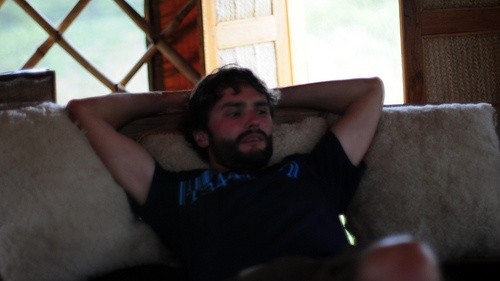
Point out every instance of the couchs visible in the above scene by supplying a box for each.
[1,96,500,281]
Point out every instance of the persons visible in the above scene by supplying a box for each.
[65,62,443,280]
